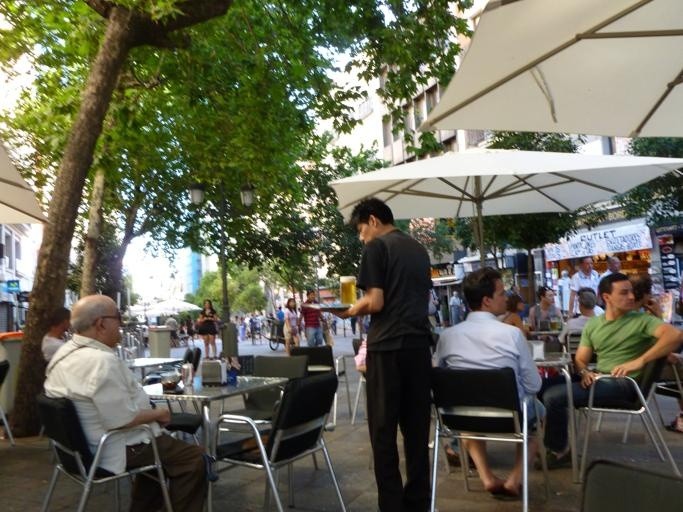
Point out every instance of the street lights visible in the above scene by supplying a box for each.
[187,174,254,358]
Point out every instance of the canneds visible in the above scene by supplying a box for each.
[181,362,194,386]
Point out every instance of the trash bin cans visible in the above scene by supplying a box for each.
[148,325,174,357]
[0,332,25,421]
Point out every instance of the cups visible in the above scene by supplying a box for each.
[550,315,560,332]
[160,371,179,392]
[339,276,356,303]
[522,317,529,330]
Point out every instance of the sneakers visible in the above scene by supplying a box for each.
[535,447,572,470]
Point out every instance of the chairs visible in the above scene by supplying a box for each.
[576,355,683,484]
[217,355,322,504]
[427,364,552,512]
[218,370,349,512]
[32,395,174,511]
[288,344,351,431]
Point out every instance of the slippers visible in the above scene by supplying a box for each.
[489,483,523,501]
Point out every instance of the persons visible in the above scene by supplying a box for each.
[41,288,371,361]
[531,272,682,472]
[662,390,682,438]
[430,266,547,497]
[428,257,682,361]
[326,196,432,512]
[42,295,209,511]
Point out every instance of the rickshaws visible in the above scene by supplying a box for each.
[257,318,294,350]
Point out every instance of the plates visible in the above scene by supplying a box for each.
[661,245,677,288]
[304,300,347,312]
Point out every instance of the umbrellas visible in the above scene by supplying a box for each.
[326,146,682,269]
[411,1,683,142]
